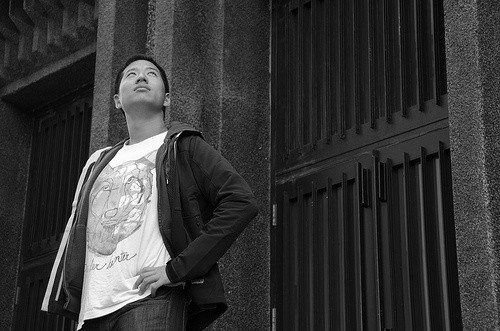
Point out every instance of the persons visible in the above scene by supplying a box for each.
[42,55,257,331]
